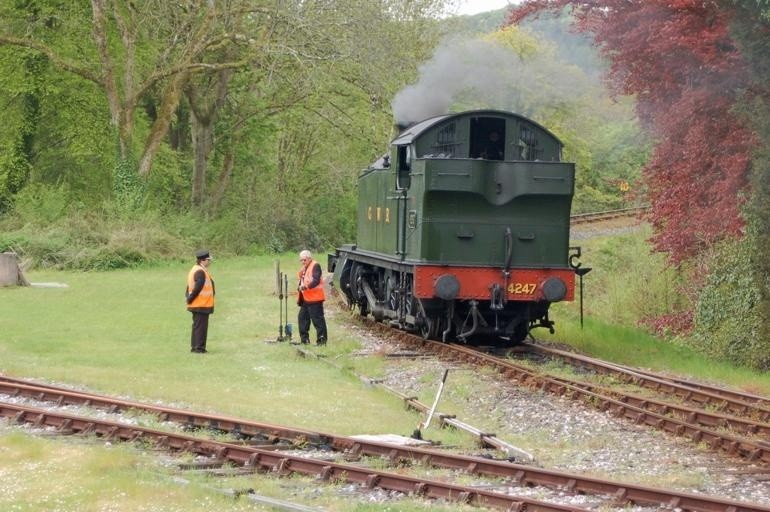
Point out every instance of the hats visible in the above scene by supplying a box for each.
[195,250,213,260]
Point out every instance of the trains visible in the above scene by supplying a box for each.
[324,110,593,345]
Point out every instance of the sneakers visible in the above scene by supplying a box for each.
[191,349,208,353]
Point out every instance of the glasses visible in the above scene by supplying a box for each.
[300,259,306,261]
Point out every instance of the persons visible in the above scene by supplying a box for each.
[183,249,216,354]
[294,249,328,346]
